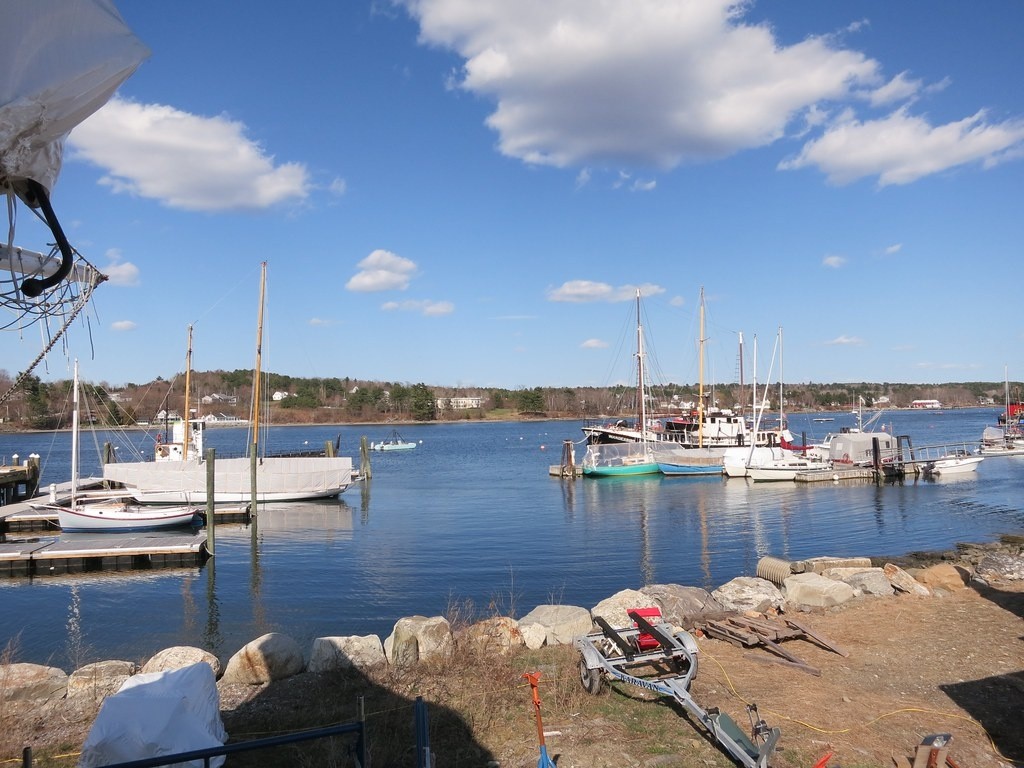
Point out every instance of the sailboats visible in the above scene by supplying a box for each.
[979,362,1024,457]
[652,286,800,475]
[580,286,794,448]
[851,390,858,414]
[103,260,352,502]
[25,358,205,533]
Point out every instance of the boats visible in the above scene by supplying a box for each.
[996,401,1024,427]
[147,420,341,458]
[374,429,416,450]
[813,418,834,421]
[744,427,897,482]
[583,442,685,476]
[867,435,985,474]
